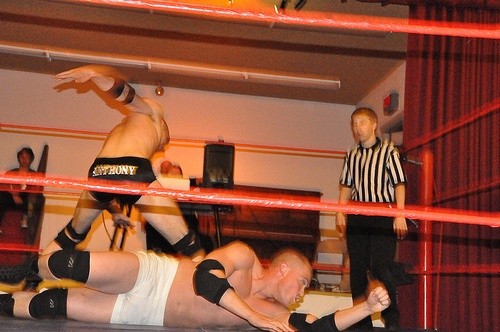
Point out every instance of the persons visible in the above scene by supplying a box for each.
[0,240,392,332]
[0,66,212,261]
[336,106,409,332]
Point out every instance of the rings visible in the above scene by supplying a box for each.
[387,299,390,303]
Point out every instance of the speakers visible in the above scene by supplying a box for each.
[202,144,235,190]
